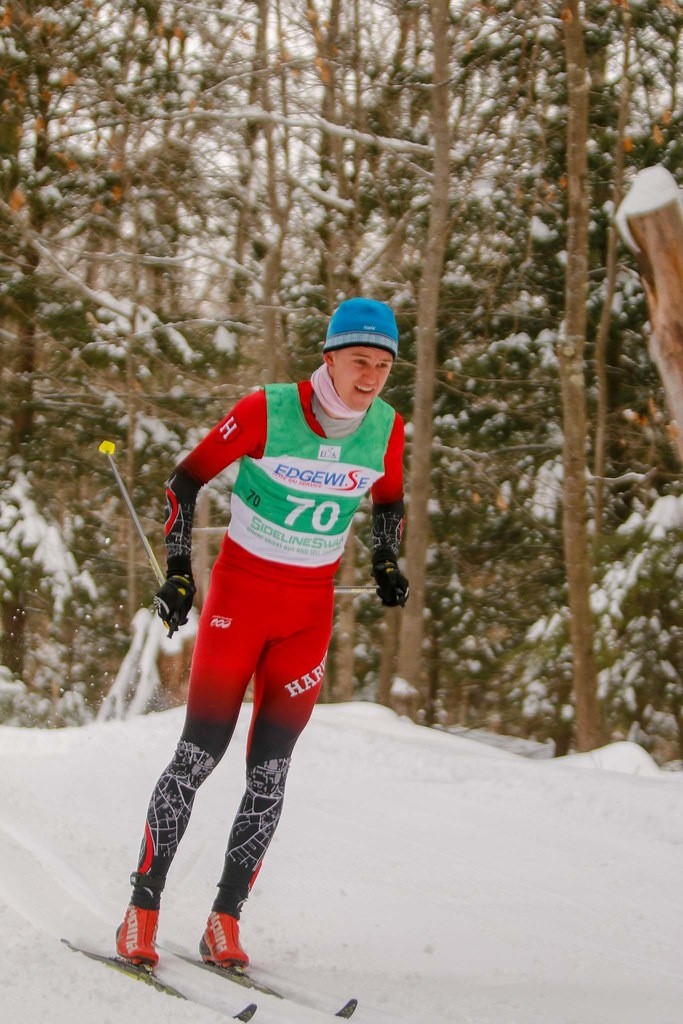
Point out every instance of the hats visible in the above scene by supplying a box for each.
[322,298,398,361]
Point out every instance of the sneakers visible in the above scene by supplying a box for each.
[116,903,160,968]
[200,911,249,969]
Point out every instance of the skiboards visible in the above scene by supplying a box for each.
[61,933,359,1024]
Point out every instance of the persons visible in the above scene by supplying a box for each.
[114,296,412,966]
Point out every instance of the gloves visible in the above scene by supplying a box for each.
[153,574,197,633]
[369,560,410,608]
